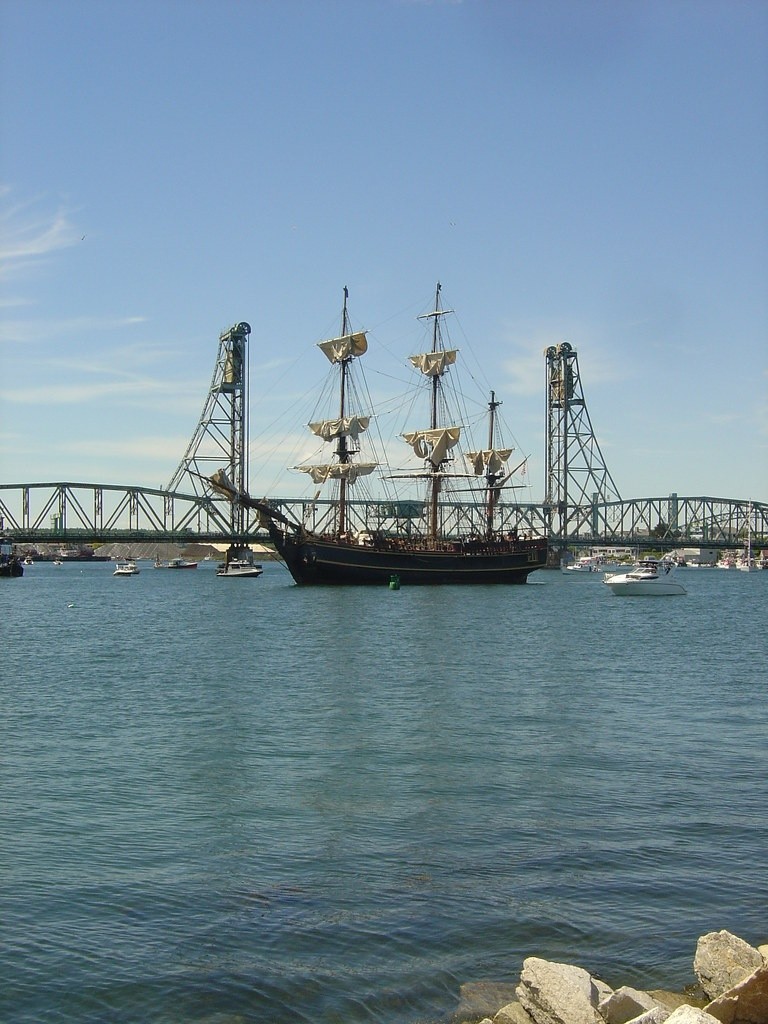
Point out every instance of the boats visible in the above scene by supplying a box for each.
[602,560,687,595]
[567,556,616,573]
[20,553,265,577]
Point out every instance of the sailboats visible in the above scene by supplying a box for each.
[717,497,760,572]
[184,280,550,585]
